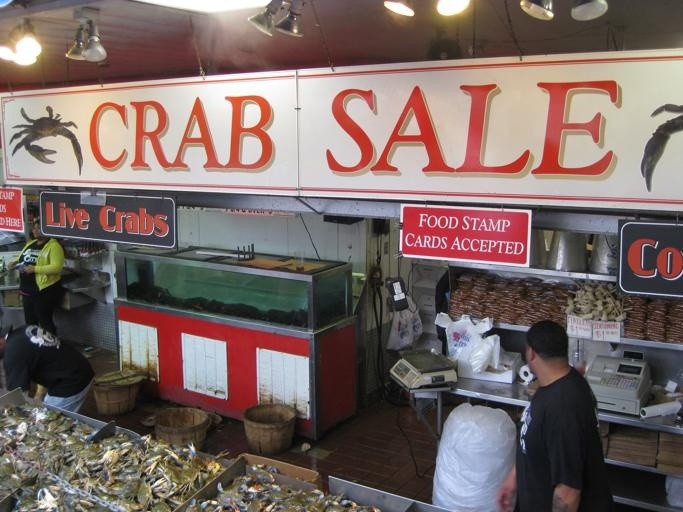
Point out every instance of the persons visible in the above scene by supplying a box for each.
[434,266,468,357]
[0,326,94,413]
[494,320,614,512]
[8,219,64,336]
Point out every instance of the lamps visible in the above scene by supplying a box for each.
[247,0,306,38]
[382,0,471,19]
[0,19,43,66]
[520,0,609,21]
[64,7,108,62]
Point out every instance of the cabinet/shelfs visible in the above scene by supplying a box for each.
[446,258,683,354]
[432,372,683,511]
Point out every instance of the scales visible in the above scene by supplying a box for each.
[389,351,458,389]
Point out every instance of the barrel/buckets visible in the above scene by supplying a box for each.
[94,382,139,415]
[244,404,297,456]
[155,407,209,451]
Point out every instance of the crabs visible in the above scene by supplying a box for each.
[9,105,84,176]
[187,462,380,512]
[640,103,683,192]
[0,400,228,512]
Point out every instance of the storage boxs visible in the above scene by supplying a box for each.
[169,451,324,511]
[457,346,522,384]
